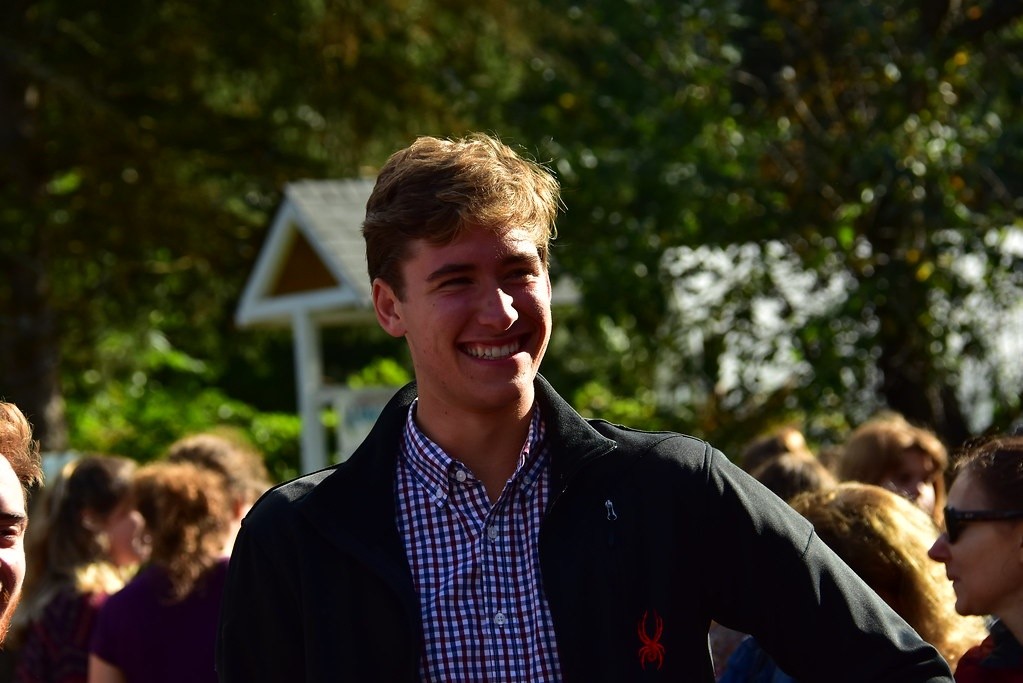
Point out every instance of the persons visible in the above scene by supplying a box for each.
[0,402,271,683]
[711,409,1023,683]
[214,131,954,683]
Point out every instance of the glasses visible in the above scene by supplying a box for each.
[943,505,1022,544]
